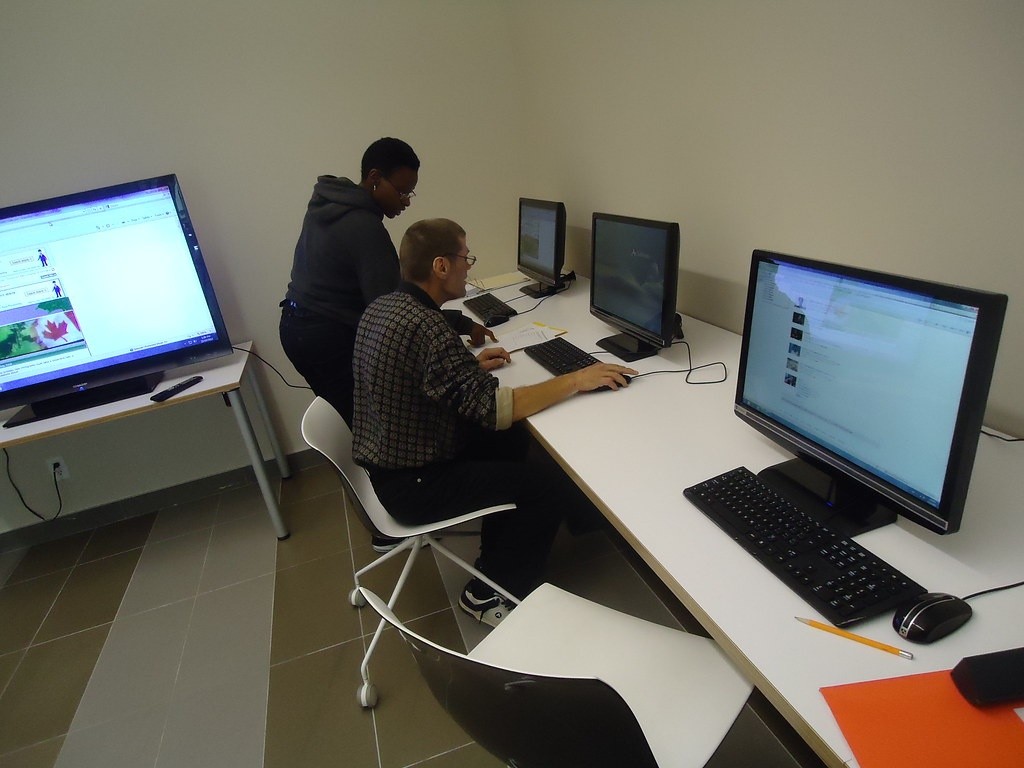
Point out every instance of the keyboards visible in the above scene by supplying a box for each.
[463,293,517,322]
[524,337,603,376]
[683,465,928,626]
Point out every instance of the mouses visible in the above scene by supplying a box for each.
[598,375,630,391]
[485,315,509,328]
[891,592,973,645]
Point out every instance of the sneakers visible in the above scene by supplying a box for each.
[457,577,517,628]
[371,536,443,552]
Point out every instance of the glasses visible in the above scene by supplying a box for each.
[443,252,477,266]
[381,174,417,200]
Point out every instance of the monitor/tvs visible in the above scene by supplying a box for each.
[589,212,680,362]
[0,173,233,429]
[733,249,1008,538]
[517,197,568,299]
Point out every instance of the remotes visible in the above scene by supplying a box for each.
[150,376,203,402]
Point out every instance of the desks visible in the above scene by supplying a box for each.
[0,340,291,542]
[438,267,1024,768]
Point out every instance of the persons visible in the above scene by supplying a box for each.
[279,136,440,554]
[352,219,639,630]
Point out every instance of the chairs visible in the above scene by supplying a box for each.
[358,582,754,768]
[302,396,522,708]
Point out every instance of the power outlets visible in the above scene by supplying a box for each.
[52,460,69,481]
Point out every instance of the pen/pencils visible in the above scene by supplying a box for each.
[794,616,914,660]
